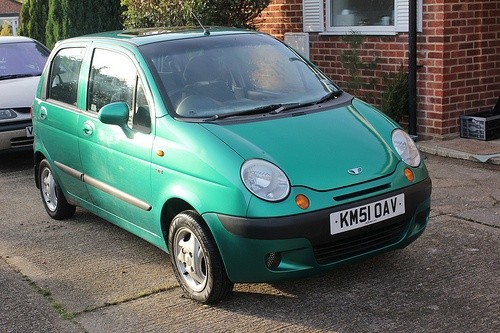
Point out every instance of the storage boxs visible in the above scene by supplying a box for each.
[460,109,500,141]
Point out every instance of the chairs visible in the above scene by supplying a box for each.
[183,53,238,103]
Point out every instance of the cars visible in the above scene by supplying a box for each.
[31,11,433,304]
[0,36,51,167]
